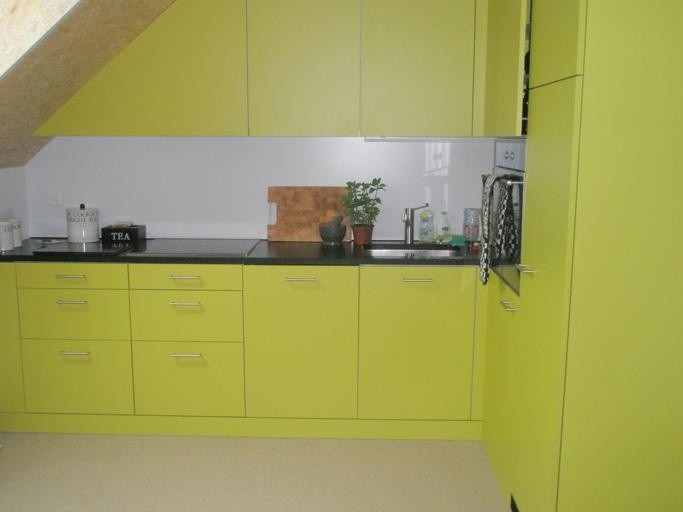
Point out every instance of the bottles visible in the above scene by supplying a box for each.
[437,211,449,235]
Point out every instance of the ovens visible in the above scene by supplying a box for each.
[486,165,527,298]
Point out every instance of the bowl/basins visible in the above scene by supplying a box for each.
[319,221,346,245]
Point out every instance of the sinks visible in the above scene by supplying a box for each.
[364,248,464,261]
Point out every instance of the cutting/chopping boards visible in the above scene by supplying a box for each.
[267,186,353,243]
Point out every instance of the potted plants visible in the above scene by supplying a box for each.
[341,177,388,247]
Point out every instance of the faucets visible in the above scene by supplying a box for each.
[405,202,429,245]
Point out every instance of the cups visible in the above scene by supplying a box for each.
[9,218,23,248]
[0,218,15,252]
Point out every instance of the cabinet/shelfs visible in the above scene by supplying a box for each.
[242,265,479,440]
[1,262,30,435]
[13,262,246,438]
[246,0,476,140]
[472,3,683,512]
[33,0,248,139]
